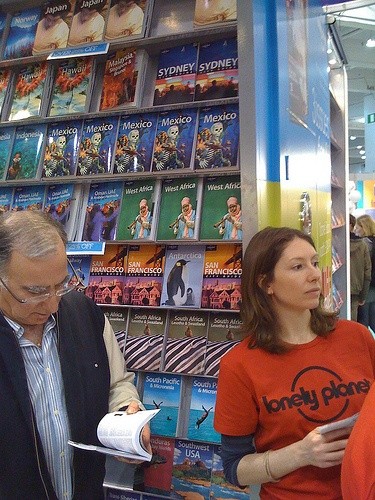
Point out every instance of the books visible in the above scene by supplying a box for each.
[67,408,160,462]
[0,0,250,500]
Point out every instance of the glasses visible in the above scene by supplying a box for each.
[2,259,83,305]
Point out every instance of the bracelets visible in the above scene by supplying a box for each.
[262,449,280,482]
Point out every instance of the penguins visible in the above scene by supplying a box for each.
[161,259,196,306]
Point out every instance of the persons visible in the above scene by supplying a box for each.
[131,198,153,239]
[32,0,71,55]
[68,0,107,45]
[219,197,243,240]
[0,210,166,469]
[173,197,196,239]
[350,214,371,321]
[213,227,375,500]
[355,215,375,333]
[105,0,144,40]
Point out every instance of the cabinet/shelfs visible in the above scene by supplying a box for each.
[1,0,349,499]
